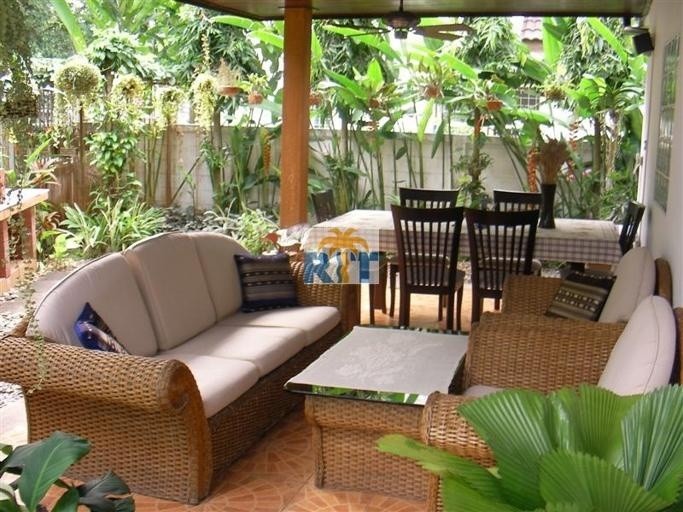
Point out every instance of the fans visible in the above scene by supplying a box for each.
[331,8,473,44]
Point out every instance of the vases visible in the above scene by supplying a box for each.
[538,183,557,229]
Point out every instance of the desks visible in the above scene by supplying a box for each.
[303,208,623,284]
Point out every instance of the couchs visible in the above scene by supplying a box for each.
[420,296,683,511]
[478,248,674,329]
[1,234,360,504]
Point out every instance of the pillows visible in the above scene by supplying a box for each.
[234,251,300,313]
[546,269,619,322]
[73,302,133,355]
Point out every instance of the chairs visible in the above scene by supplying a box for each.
[558,199,646,278]
[391,207,466,330]
[311,189,337,223]
[492,190,544,215]
[397,185,460,208]
[469,207,541,327]
